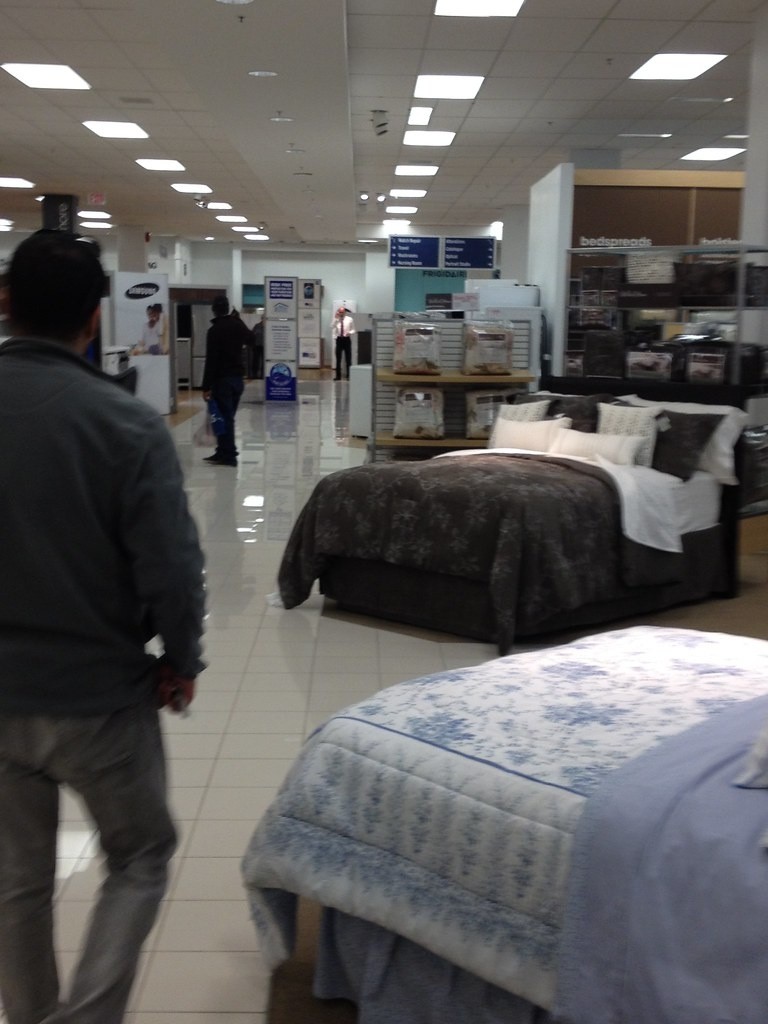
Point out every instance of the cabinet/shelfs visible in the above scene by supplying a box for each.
[364,314,545,460]
[565,244,768,516]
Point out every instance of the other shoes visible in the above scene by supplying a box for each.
[333,376,341,380]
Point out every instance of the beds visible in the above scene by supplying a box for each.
[275,388,754,658]
[235,622,767,1024]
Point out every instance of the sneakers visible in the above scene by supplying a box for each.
[207,458,238,466]
[202,454,221,460]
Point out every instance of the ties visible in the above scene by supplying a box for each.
[340,318,344,337]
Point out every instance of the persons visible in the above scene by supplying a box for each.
[200,294,255,467]
[0,226,207,1024]
[248,313,266,378]
[329,307,355,382]
[139,302,167,354]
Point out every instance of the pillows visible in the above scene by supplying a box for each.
[595,400,663,471]
[652,407,727,482]
[546,428,647,470]
[487,399,550,451]
[493,414,574,456]
[513,392,618,435]
